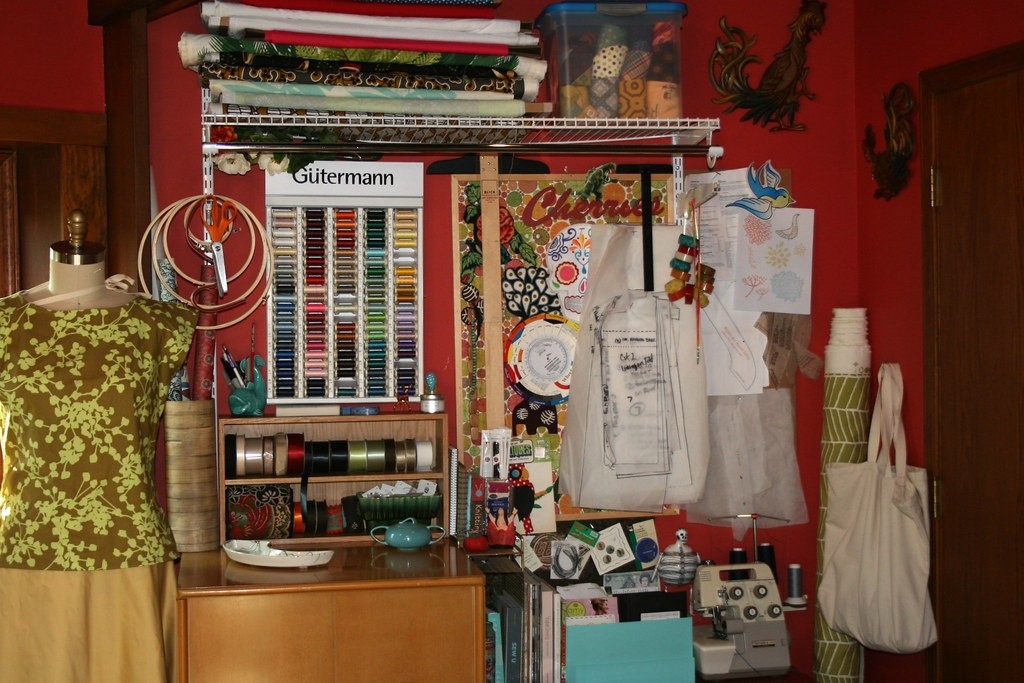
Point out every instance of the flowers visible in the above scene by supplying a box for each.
[209,125,289,175]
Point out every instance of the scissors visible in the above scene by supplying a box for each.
[200,193,238,297]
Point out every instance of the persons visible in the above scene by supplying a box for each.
[0,244,197,683]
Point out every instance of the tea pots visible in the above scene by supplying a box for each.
[369,517,446,551]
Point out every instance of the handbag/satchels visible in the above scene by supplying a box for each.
[816,364,938,654]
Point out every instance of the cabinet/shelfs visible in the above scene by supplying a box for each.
[218,413,449,547]
[175,550,488,683]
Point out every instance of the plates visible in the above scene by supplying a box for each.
[222,539,334,568]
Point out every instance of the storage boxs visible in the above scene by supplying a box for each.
[533,0,688,125]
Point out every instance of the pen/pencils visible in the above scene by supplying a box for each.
[221,321,256,392]
